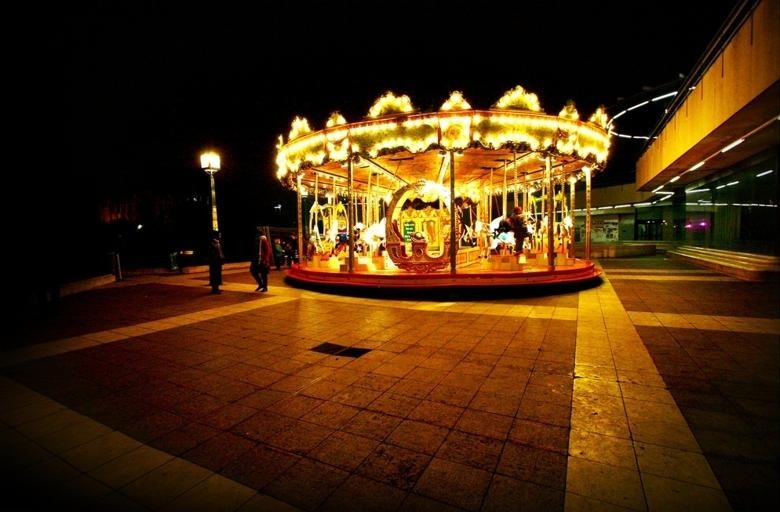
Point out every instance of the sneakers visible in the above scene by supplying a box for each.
[256,285,267,292]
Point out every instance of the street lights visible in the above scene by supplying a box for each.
[200,152,221,232]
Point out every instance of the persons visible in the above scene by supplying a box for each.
[510,207,533,254]
[249,225,307,292]
[205,231,224,295]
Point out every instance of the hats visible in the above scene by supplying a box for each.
[513,207,522,214]
